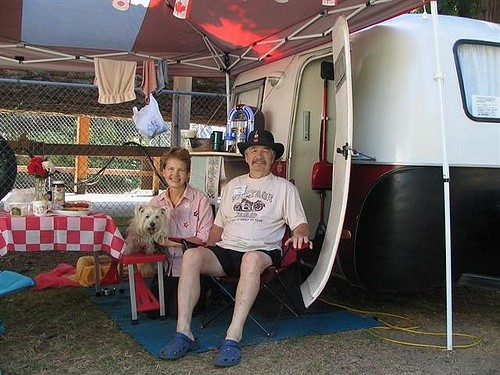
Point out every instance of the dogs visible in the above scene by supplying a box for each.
[117,204,172,281]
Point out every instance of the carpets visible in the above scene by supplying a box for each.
[83,277,384,361]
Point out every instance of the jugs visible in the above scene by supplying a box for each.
[210,131,223,151]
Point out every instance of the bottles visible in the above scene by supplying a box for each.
[224,137,236,152]
[52,181,65,207]
[235,128,246,154]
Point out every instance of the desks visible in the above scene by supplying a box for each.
[4,193,161,222]
[0,209,125,296]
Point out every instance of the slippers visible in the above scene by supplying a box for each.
[215,339,241,366]
[160,331,200,359]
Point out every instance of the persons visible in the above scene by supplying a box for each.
[150,147,214,321]
[159,130,314,367]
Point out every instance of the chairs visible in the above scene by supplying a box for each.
[179,180,309,339]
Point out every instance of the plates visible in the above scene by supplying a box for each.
[62,201,94,210]
[54,210,93,216]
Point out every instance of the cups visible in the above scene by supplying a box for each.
[32,200,52,216]
[10,203,31,216]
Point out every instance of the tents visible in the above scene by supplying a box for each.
[0,1,452,351]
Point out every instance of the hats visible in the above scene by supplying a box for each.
[237,129,284,160]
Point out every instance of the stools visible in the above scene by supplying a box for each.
[119,250,166,325]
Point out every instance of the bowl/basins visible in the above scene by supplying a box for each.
[180,130,198,138]
[3,202,24,212]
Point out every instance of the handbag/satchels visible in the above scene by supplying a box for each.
[131,93,169,141]
